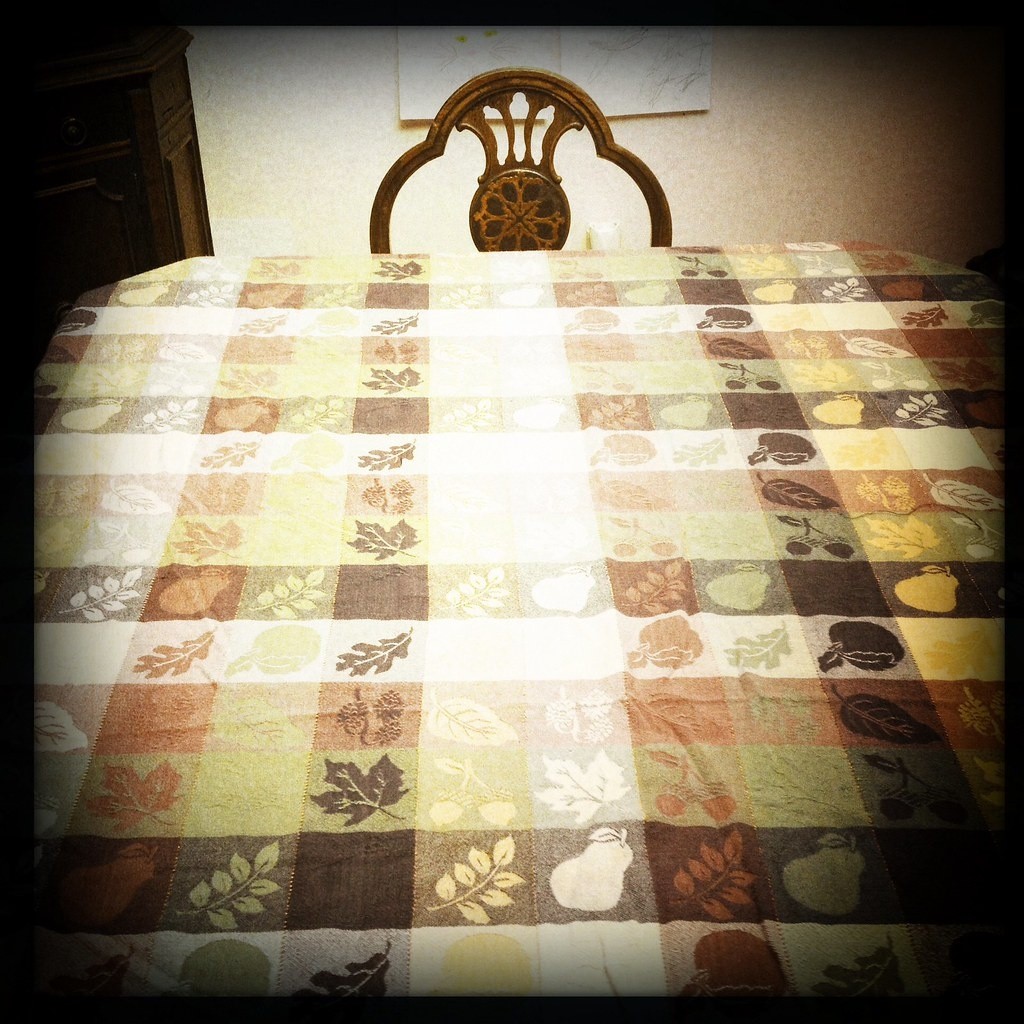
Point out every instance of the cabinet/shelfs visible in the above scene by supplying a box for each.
[8,23,218,356]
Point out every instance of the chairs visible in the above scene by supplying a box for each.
[369,69,673,253]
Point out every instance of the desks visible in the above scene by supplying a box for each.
[31,240,1007,996]
